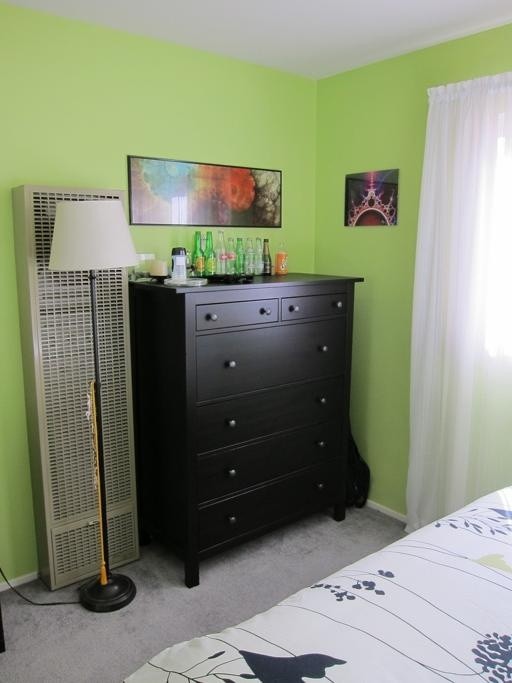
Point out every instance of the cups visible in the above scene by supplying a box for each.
[139,255,168,277]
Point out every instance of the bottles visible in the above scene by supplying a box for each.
[171,246,187,281]
[275,240,289,276]
[187,229,272,276]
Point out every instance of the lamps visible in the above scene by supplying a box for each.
[49,197,140,613]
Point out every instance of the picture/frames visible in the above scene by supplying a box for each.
[127,154,282,226]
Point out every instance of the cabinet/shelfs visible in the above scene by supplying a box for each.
[128,266,369,587]
